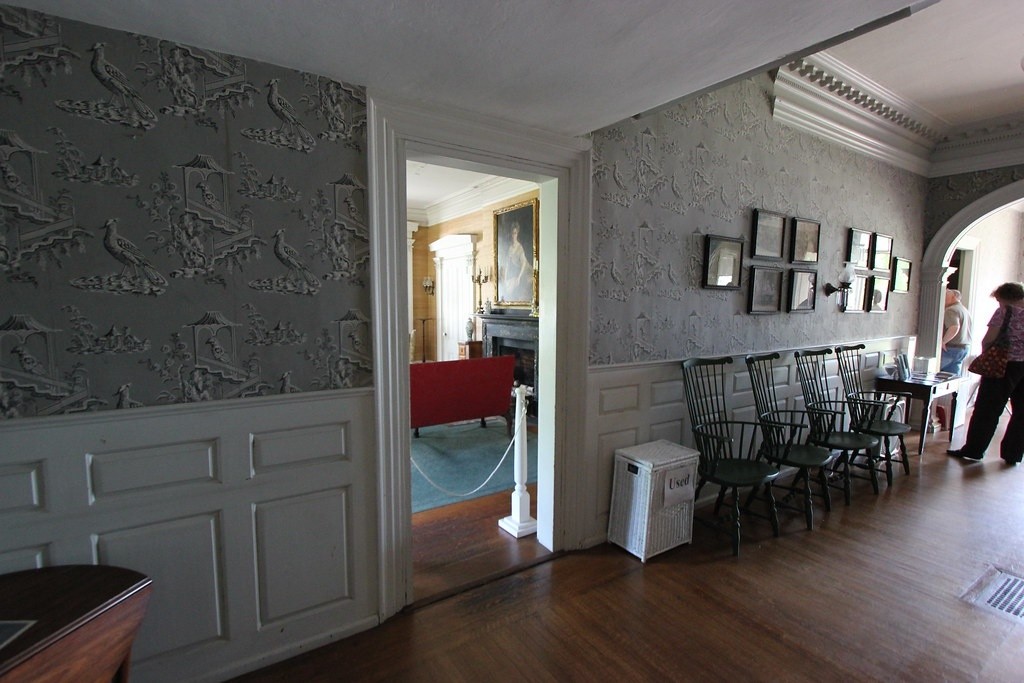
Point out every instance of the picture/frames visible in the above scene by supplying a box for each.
[847,228,872,269]
[839,274,868,313]
[788,270,818,313]
[791,217,821,265]
[869,275,892,312]
[492,196,540,306]
[891,257,912,294]
[703,233,744,290]
[873,232,893,271]
[753,209,787,260]
[747,266,785,315]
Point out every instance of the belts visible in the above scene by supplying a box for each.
[947,343,967,348]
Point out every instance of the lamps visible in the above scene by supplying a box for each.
[422,276,434,295]
[826,265,851,296]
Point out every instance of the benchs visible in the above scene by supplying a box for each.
[408,355,516,439]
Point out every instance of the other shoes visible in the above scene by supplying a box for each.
[946,447,982,461]
[1006,460,1016,466]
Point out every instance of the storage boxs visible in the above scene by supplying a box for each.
[607,439,705,564]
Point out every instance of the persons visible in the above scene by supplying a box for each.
[946,283,1024,466]
[940,290,973,375]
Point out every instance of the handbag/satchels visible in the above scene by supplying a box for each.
[968,305,1012,378]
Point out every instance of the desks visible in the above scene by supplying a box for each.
[870,369,960,455]
[0,559,153,683]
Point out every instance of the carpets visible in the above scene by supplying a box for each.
[410,417,537,514]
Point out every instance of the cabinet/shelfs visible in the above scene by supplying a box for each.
[458,341,483,359]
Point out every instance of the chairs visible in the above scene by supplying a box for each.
[681,355,780,557]
[834,344,911,489]
[793,347,879,507]
[745,354,834,531]
